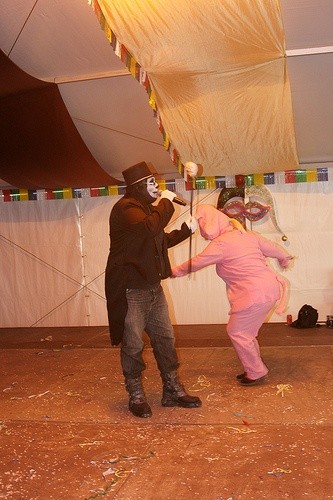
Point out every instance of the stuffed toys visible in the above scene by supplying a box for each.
[171,203,295,385]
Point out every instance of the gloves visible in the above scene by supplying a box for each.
[183,216,197,234]
[160,190,176,203]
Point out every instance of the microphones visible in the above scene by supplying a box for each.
[157,190,186,206]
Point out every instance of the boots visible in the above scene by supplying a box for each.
[123,375,152,418]
[161,369,201,409]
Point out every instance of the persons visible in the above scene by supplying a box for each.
[105,161,202,418]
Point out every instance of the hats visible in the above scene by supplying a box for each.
[121,161,154,190]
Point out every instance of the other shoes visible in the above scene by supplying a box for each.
[237,372,247,379]
[238,373,268,386]
